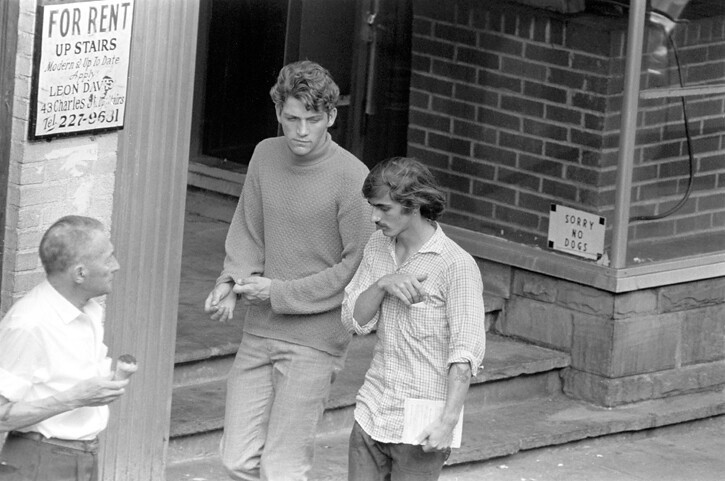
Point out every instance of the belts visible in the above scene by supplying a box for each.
[11,430,100,454]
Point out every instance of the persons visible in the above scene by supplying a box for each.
[339,158,486,481]
[0,214,129,481]
[203,61,377,481]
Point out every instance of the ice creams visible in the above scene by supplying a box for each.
[115,353,138,380]
